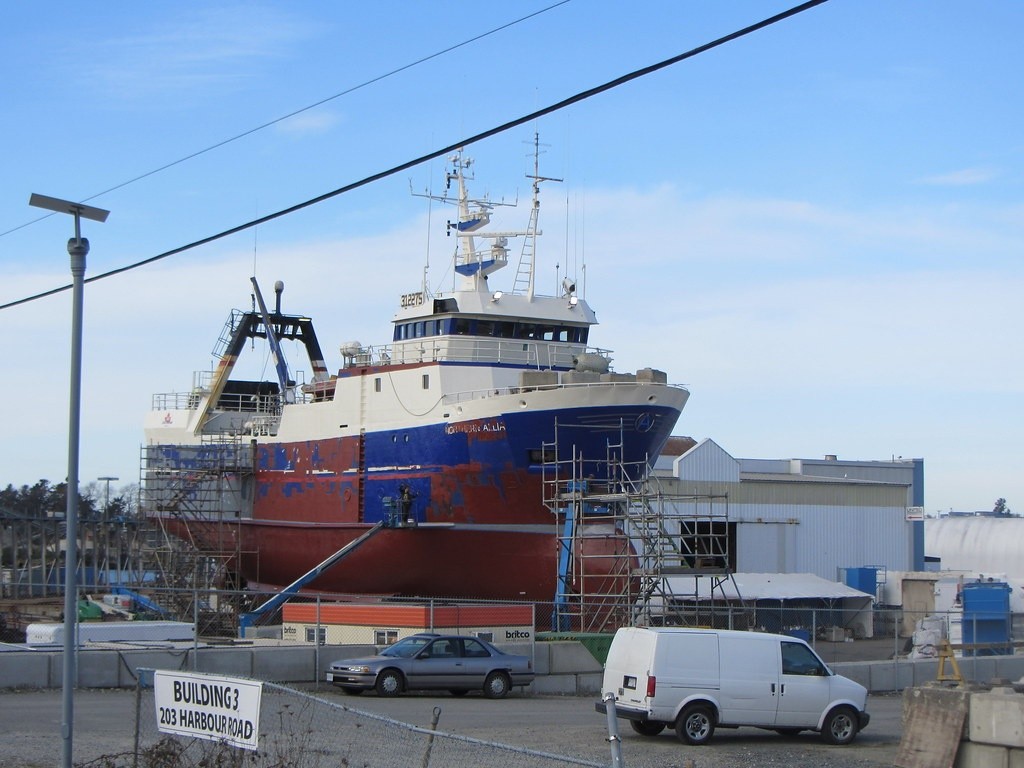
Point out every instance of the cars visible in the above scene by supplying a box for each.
[324,633,536,700]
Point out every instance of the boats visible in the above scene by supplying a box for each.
[135,127,692,625]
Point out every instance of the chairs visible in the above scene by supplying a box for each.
[445,645,453,656]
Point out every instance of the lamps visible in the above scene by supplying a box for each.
[567,297,578,309]
[491,291,502,302]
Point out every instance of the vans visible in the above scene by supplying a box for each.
[594,628,870,745]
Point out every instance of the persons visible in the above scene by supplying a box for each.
[399,484,419,521]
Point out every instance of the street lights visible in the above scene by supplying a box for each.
[28,193,113,768]
[97,477,119,589]
